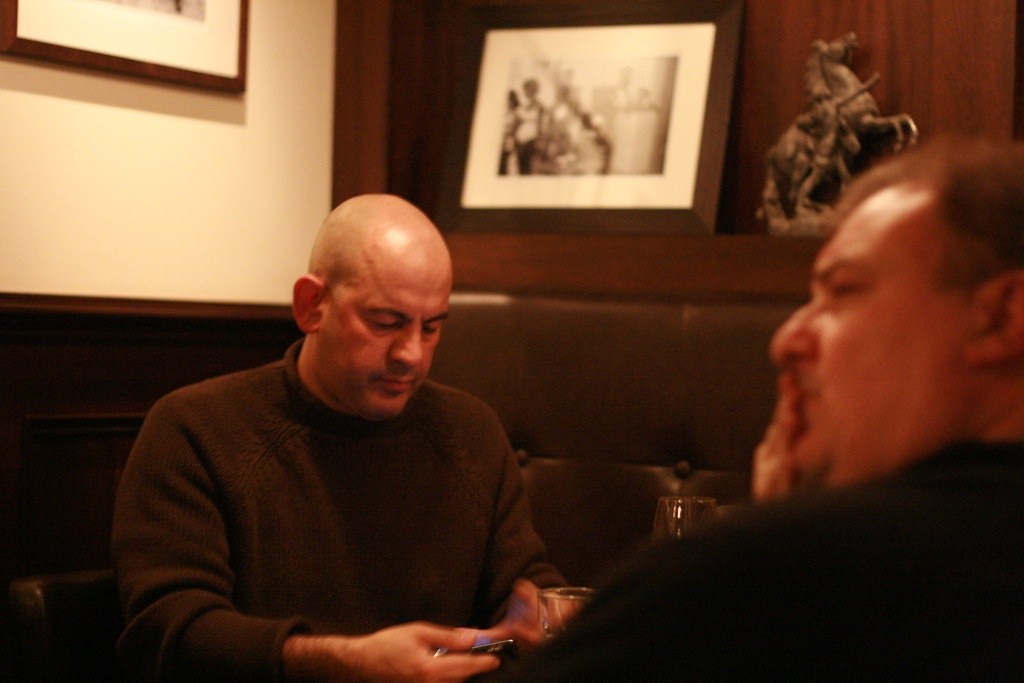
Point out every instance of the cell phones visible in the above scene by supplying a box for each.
[469,639,517,655]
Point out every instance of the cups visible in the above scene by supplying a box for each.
[537,588,595,642]
[652,496,718,549]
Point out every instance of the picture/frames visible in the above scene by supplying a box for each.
[0,0,248,92]
[417,0,746,235]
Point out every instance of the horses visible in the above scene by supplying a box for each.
[762,30,919,219]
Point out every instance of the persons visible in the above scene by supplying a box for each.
[795,73,881,208]
[499,80,612,175]
[471,143,1024,683]
[108,195,579,683]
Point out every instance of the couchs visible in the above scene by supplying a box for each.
[8,297,799,683]
